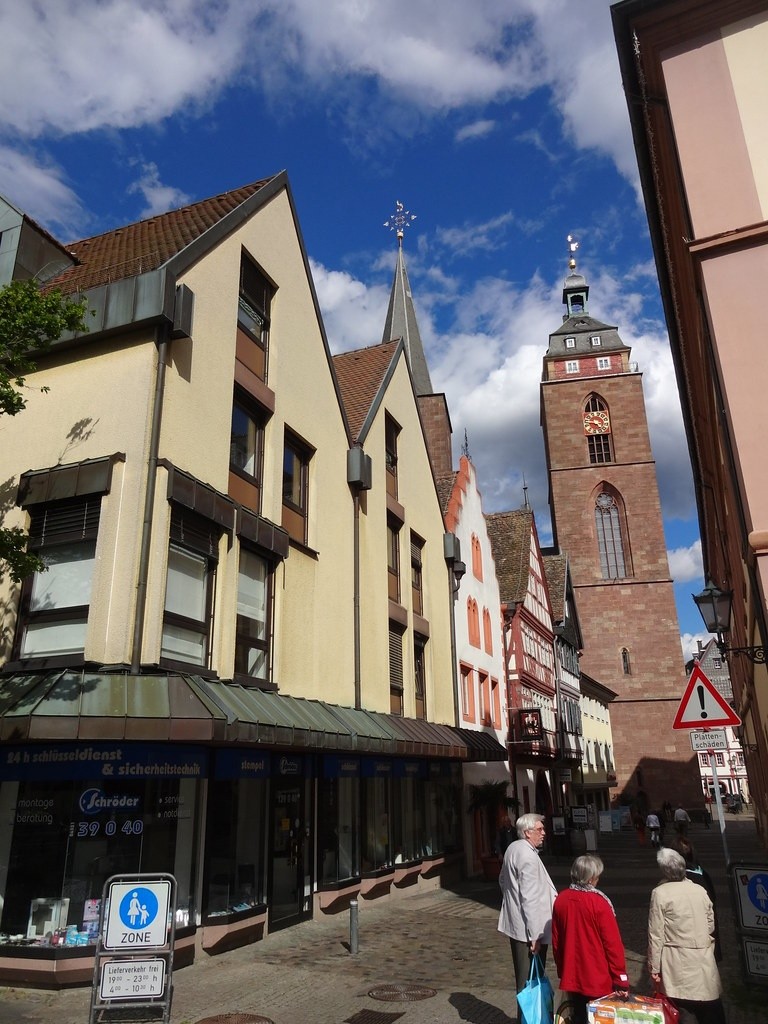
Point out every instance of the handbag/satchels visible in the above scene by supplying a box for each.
[648,981,680,1024]
[516,953,555,1024]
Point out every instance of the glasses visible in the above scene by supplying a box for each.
[528,827,545,833]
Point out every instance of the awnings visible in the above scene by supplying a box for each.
[0,672,509,763]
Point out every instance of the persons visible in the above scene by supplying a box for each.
[644,808,667,847]
[674,803,692,837]
[552,855,629,1024]
[497,813,559,1024]
[646,836,726,1024]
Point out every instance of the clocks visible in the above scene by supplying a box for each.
[582,410,611,436]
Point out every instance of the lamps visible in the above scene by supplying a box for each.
[690,572,768,665]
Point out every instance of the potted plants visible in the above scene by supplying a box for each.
[467,777,524,882]
